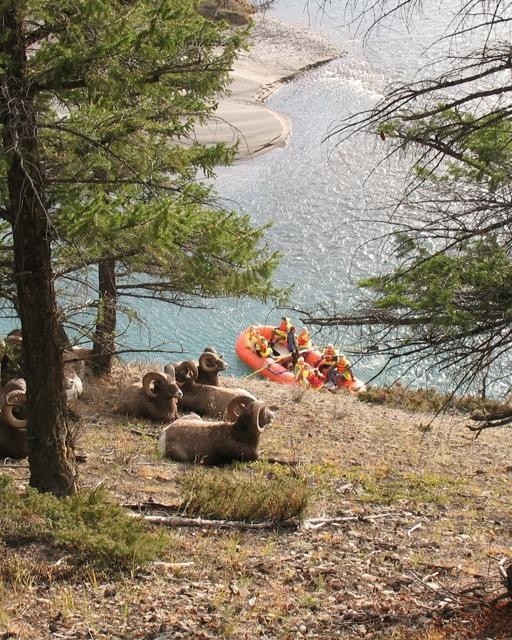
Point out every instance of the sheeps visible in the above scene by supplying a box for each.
[0,328,93,401]
[157,392,276,466]
[163,359,254,421]
[0,376,89,464]
[120,363,184,420]
[190,346,229,387]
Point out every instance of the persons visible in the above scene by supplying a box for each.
[245,317,356,389]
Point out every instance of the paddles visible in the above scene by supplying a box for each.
[243,345,319,380]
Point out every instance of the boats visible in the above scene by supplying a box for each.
[238,325,366,393]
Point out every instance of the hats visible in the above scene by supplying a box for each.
[297,357,304,361]
